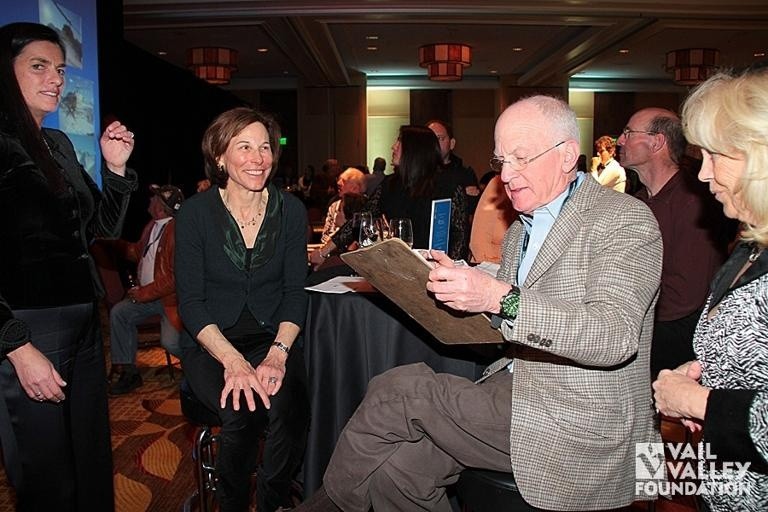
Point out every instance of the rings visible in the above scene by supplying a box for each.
[129,131,135,140]
[269,377,278,384]
[34,388,43,400]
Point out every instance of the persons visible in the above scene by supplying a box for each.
[588,137,630,195]
[1,26,134,511]
[296,94,664,512]
[647,69,768,512]
[174,105,310,511]
[615,107,720,333]
[100,121,510,401]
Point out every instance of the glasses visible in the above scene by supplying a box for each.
[622,126,655,139]
[490,138,563,172]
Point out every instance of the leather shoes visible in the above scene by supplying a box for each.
[106,371,144,395]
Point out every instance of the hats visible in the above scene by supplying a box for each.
[149,184,184,211]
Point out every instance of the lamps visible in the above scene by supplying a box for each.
[664,49,732,92]
[417,44,474,82]
[189,46,240,86]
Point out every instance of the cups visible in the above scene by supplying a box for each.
[358,217,414,251]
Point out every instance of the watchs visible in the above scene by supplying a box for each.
[268,338,290,359]
[490,282,519,331]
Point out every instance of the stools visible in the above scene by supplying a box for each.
[468,467,517,511]
[136,325,175,384]
[646,414,703,512]
[179,394,221,512]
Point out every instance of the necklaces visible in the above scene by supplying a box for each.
[221,191,270,230]
[746,245,763,263]
